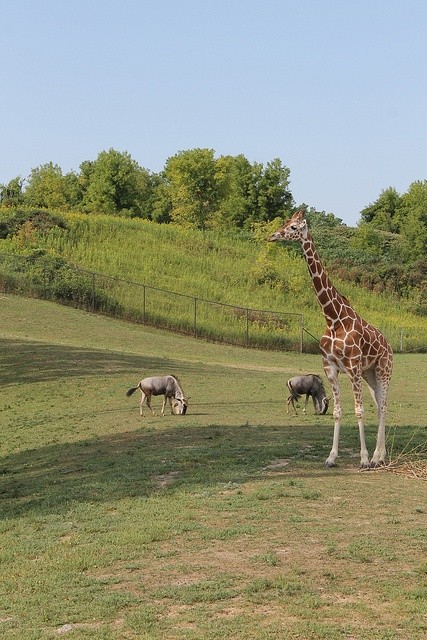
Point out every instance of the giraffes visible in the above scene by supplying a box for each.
[266,210,392,469]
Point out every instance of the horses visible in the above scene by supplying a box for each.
[126,375,191,417]
[286,374,331,415]
